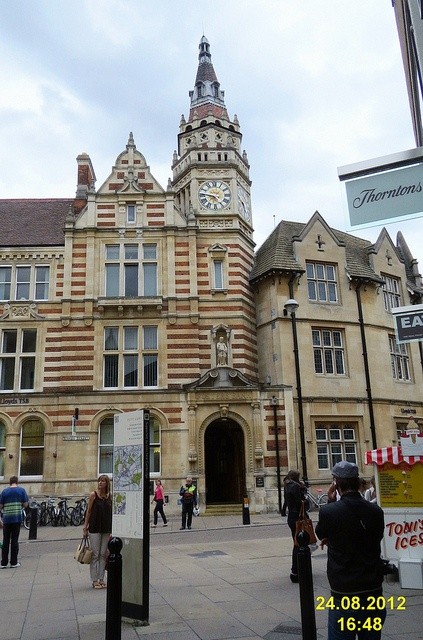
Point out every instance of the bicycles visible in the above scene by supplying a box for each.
[305,489,328,513]
[71,496,88,526]
[22,495,74,530]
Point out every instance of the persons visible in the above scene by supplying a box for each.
[364,475,377,503]
[82,474,111,590]
[216,336,227,367]
[315,460,385,639]
[151,478,167,527]
[0,476,28,569]
[179,477,197,529]
[281,467,307,581]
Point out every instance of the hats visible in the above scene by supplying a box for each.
[331,461,360,478]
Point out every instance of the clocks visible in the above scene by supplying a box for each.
[197,179,233,212]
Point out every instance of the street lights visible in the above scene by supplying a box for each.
[284,299,310,511]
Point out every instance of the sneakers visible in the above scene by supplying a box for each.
[10,562,21,568]
[0,564,7,568]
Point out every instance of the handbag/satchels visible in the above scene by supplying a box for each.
[74,537,93,564]
[294,500,318,546]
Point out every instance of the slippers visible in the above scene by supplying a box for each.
[98,580,107,587]
[92,583,102,589]
